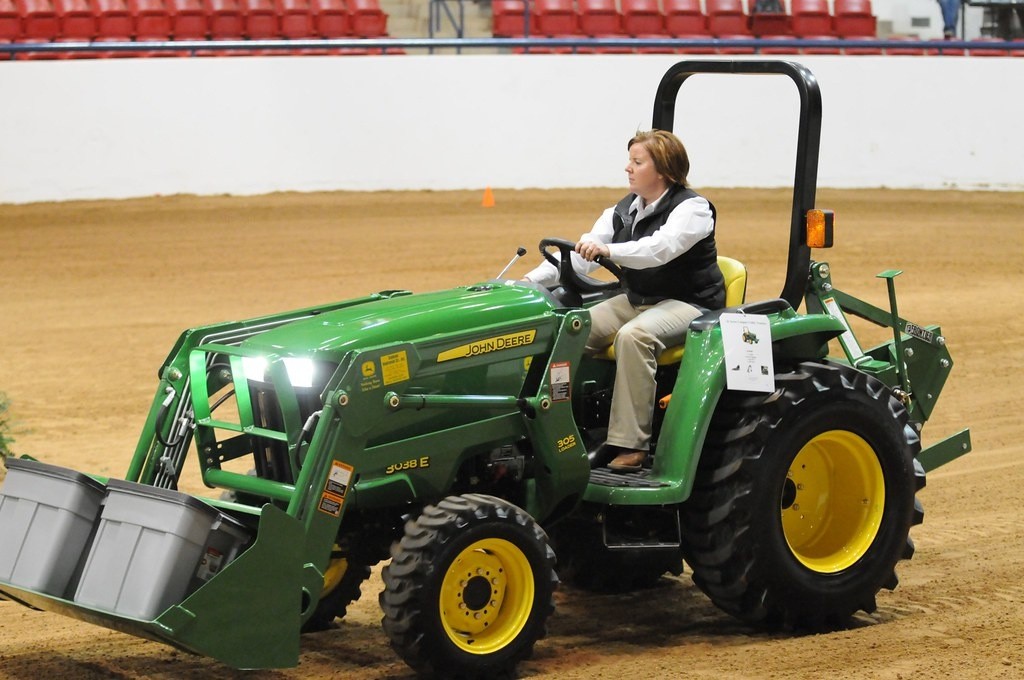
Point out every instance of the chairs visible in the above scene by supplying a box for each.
[0,0,405,61]
[591,256,747,363]
[487,0,1024,57]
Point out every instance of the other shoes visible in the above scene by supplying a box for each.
[607,448,647,471]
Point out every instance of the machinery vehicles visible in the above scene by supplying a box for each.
[0,60,972,680]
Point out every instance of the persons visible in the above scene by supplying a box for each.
[937,0,962,38]
[521,127,728,468]
[742,326,756,344]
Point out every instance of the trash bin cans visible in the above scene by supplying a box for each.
[72,477,257,622]
[1,458,110,601]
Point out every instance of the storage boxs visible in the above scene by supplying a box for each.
[1,457,254,620]
